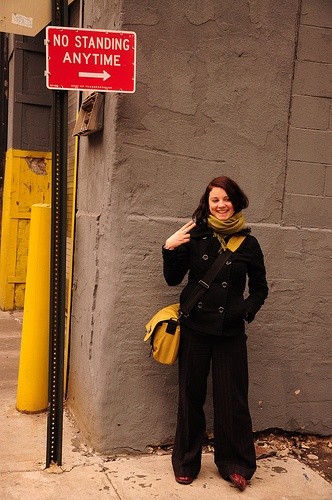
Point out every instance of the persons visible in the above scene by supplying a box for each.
[162,176,270,492]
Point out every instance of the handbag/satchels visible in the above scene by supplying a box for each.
[143,302,181,365]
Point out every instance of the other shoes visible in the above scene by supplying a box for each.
[229,472,247,492]
[176,476,193,484]
